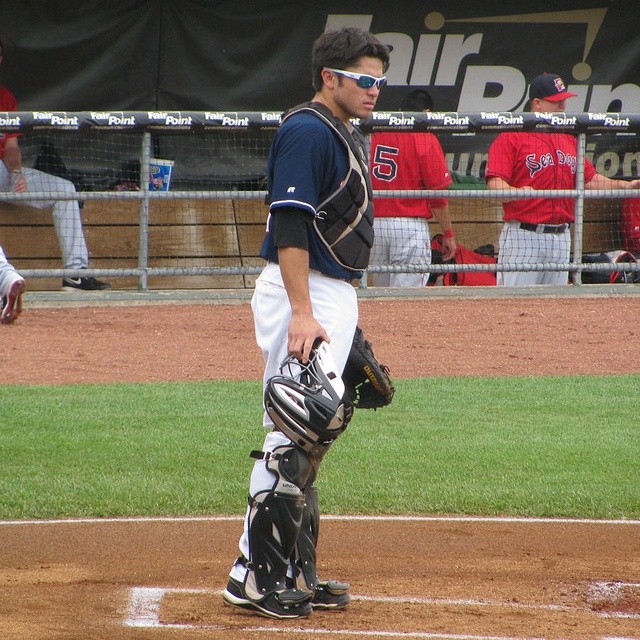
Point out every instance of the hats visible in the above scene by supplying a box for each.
[529,71,578,102]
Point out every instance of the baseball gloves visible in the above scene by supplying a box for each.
[342,327,394,409]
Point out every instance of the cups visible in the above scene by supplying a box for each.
[149,158,174,191]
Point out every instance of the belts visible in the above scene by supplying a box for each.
[519,222,571,233]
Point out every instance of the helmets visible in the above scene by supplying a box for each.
[264,338,355,459]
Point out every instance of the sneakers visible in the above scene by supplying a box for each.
[286,578,351,611]
[0,270,27,324]
[222,556,313,620]
[61,276,113,291]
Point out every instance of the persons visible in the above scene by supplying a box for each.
[0,244,26,324]
[0,41,113,292]
[620,198,640,255]
[370,90,458,286]
[222,30,391,620]
[484,72,640,286]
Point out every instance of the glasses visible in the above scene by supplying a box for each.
[322,66,387,90]
